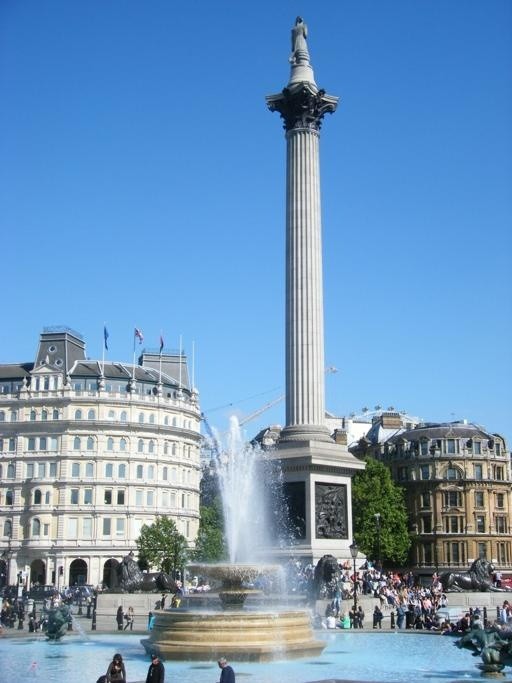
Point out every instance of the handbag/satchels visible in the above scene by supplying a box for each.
[124,615,132,620]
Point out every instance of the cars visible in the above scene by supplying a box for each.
[1,584,17,598]
[62,583,96,601]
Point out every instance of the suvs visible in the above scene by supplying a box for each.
[21,584,57,600]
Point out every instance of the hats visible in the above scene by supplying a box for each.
[151,653,158,660]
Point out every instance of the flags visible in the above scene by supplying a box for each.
[105,325,109,350]
[159,335,164,353]
[135,326,145,343]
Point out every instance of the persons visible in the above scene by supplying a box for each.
[106,653,127,682]
[145,654,165,682]
[0,574,210,630]
[314,558,511,634]
[217,657,235,683]
[291,14,308,52]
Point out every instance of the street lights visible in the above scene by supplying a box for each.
[373,511,382,569]
[172,525,179,580]
[349,537,361,627]
